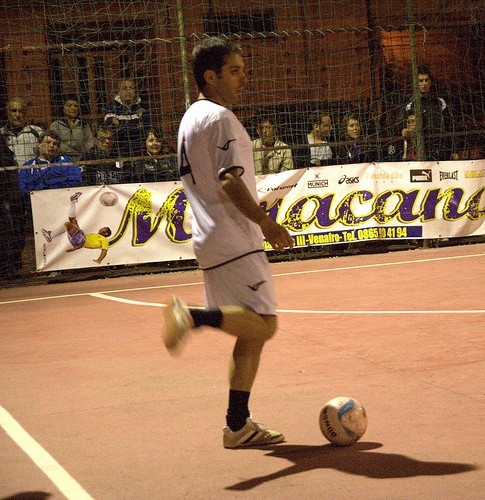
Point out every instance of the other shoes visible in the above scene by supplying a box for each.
[223,419,285,448]
[163,297,192,358]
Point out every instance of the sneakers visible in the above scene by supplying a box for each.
[42,228,52,242]
[70,192,83,201]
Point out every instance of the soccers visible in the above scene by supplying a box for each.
[320,397,367,445]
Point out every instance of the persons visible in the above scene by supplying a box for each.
[78,126,132,185]
[387,110,418,161]
[297,110,345,169]
[20,131,83,212]
[162,37,295,450]
[339,112,368,165]
[103,78,149,159]
[249,119,293,175]
[131,127,179,182]
[0,97,45,166]
[392,68,459,161]
[49,95,95,161]
